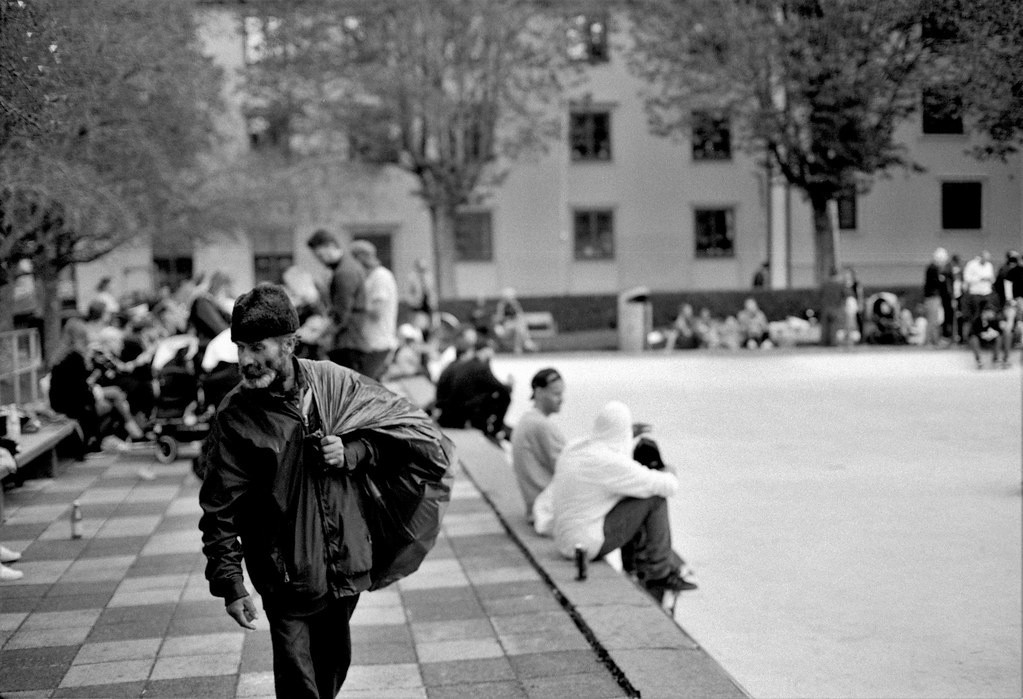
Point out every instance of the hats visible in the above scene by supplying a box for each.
[231,285,300,343]
[531,368,562,400]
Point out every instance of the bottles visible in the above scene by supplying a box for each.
[69,499,83,537]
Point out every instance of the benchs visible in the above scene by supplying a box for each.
[0,395,114,483]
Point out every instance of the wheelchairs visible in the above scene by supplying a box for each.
[151,349,214,462]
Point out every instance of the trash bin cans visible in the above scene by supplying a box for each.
[616,285,653,355]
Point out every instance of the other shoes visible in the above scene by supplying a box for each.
[0,564,24,583]
[643,569,696,594]
[124,419,144,439]
[100,434,133,453]
[0,547,22,563]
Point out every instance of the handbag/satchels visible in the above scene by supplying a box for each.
[297,354,457,590]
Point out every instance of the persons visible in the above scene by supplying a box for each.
[530,399,702,592]
[198,282,455,699]
[46,229,1023,454]
[510,365,570,527]
[0,484,23,582]
